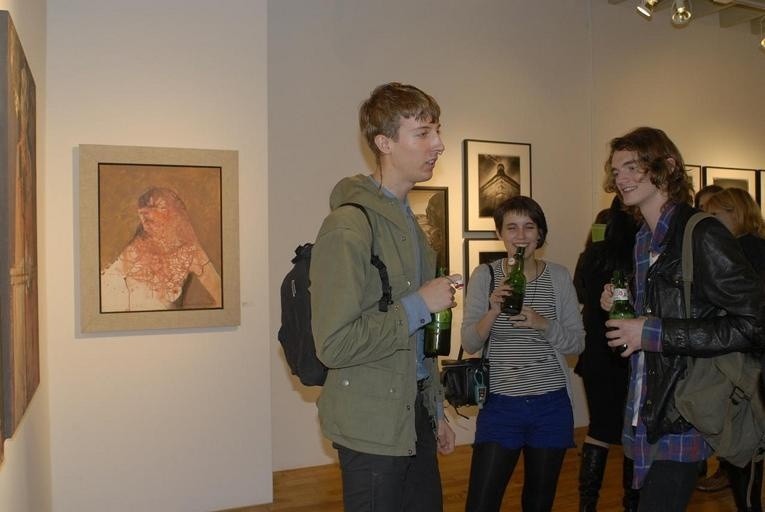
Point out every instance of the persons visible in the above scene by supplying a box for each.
[461,198,587,512]
[573,192,640,511]
[308,81,461,512]
[707,188,764,511]
[602,125,765,512]
[695,184,731,490]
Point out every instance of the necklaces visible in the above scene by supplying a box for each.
[505,258,538,309]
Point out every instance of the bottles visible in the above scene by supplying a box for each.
[609,269,637,353]
[424,266,452,357]
[500,247,525,314]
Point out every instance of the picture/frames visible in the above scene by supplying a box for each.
[463,139,532,232]
[407,186,449,278]
[78,143,241,333]
[0,10,40,438]
[682,164,765,220]
[465,238,508,298]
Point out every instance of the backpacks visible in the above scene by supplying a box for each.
[277,202,394,387]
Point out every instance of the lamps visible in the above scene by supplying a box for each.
[671,0,692,22]
[637,0,659,17]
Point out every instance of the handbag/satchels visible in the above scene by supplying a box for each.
[440,356,491,420]
[673,350,764,486]
